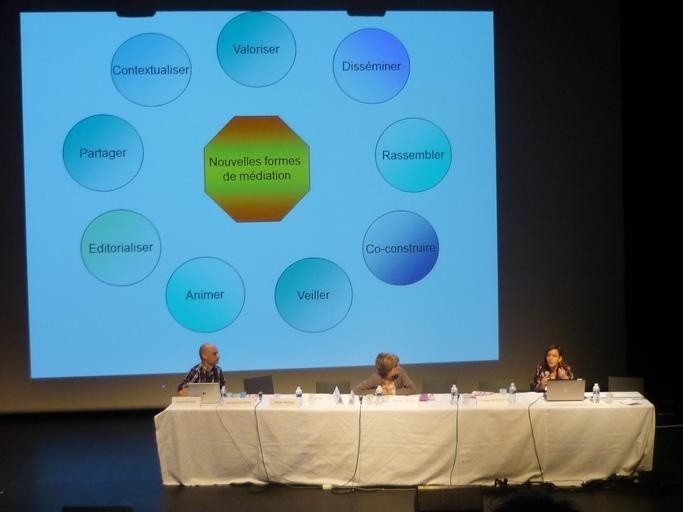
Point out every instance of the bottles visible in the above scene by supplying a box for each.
[220,385,227,406]
[295,386,303,407]
[451,384,458,406]
[375,385,384,406]
[508,382,516,405]
[593,382,600,406]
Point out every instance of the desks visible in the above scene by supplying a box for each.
[154,392,656,487]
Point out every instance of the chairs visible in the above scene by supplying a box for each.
[608,377,644,391]
[315,380,352,394]
[421,379,458,392]
[244,374,274,394]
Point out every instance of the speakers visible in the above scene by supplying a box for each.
[415,486,483,512]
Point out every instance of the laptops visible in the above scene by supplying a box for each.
[188,383,221,403]
[544,379,585,401]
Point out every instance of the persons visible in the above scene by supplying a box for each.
[531,346,575,392]
[353,352,415,395]
[177,342,226,395]
